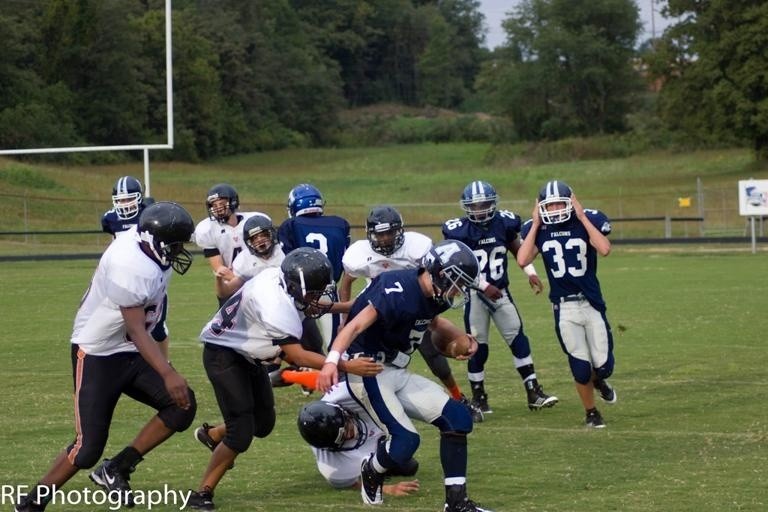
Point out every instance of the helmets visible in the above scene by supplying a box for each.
[281,248,335,319]
[460,180,498,224]
[423,240,480,309]
[538,180,574,225]
[206,184,240,222]
[137,201,194,274]
[242,215,277,256]
[287,184,324,218]
[366,207,404,256]
[112,176,143,221]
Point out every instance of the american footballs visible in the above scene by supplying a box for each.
[432,326,470,357]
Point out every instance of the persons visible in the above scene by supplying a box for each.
[100,175,169,363]
[188,183,495,512]
[12,200,196,511]
[441,180,559,415]
[517,180,617,429]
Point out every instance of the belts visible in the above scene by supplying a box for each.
[555,294,584,304]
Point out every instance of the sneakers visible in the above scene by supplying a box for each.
[528,391,558,411]
[268,365,314,398]
[89,458,136,509]
[595,379,617,403]
[361,453,382,506]
[186,489,215,511]
[450,392,493,423]
[587,414,606,428]
[193,423,234,470]
[443,498,492,512]
